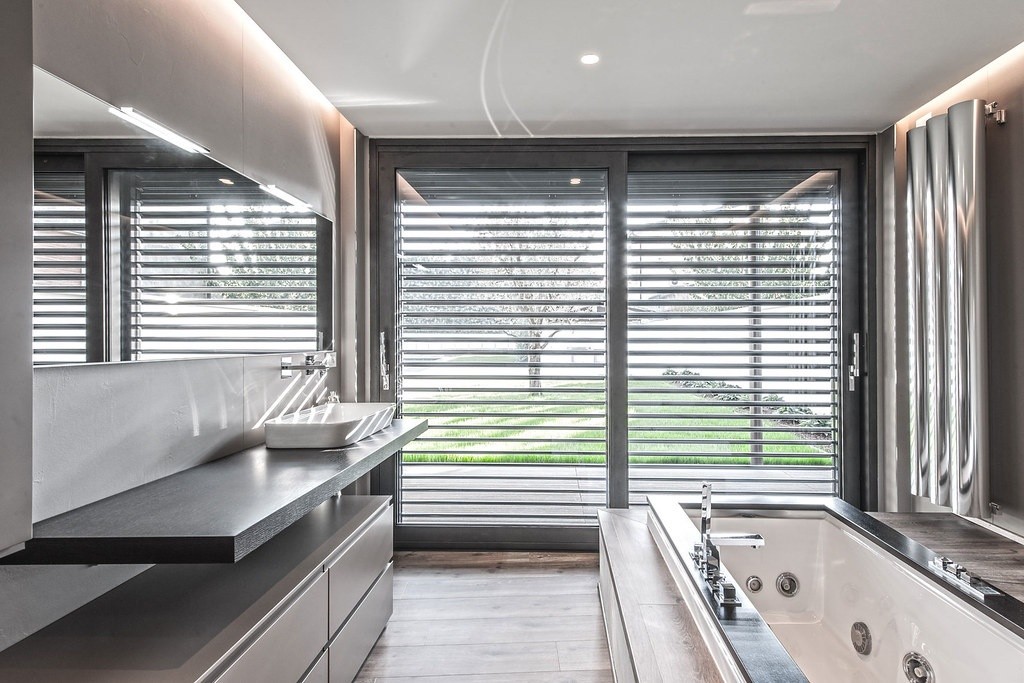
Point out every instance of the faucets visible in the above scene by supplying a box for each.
[703,534,766,580]
[280,357,325,380]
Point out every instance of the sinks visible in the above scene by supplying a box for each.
[265,403,396,448]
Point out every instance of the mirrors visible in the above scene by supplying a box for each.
[33,64,333,369]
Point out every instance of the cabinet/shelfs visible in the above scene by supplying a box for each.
[0,419,428,682]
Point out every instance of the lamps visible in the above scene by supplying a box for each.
[267,184,312,207]
[108,107,198,153]
[121,107,210,154]
[256,185,306,207]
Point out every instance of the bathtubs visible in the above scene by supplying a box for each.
[597,494,1023,683]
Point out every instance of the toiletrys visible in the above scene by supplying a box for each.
[328,391,339,403]
